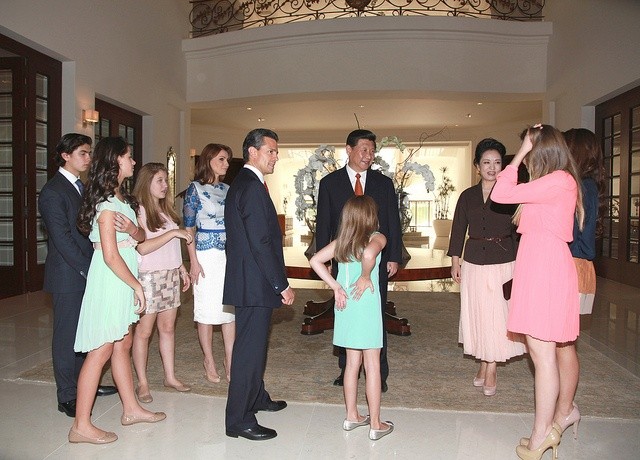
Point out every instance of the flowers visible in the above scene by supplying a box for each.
[293,112,456,233]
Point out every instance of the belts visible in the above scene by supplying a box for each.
[468,235,512,241]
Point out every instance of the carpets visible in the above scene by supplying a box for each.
[15,290,639,420]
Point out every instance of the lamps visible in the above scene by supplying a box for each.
[84,110,99,124]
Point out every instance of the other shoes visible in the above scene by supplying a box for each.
[68,426,117,444]
[369,421,394,440]
[121,408,167,426]
[164,377,192,392]
[343,414,370,431]
[135,385,153,403]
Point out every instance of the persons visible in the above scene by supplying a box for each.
[224,129,296,441]
[68,135,167,445]
[37,132,119,420]
[489,125,582,460]
[563,129,609,410]
[131,162,193,403]
[308,195,394,441]
[315,129,401,393]
[446,138,529,396]
[184,143,233,383]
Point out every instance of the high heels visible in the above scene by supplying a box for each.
[473,377,485,387]
[223,358,230,382]
[482,372,497,396]
[203,360,221,383]
[555,401,582,439]
[515,422,562,460]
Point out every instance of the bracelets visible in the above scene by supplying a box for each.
[281,285,290,294]
[179,272,189,277]
[129,227,140,237]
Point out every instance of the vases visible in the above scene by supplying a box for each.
[433,238,449,249]
[434,220,450,236]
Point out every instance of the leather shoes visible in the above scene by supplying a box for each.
[253,400,287,415]
[381,380,388,392]
[226,424,278,440]
[58,399,91,418]
[95,385,117,396]
[333,372,360,386]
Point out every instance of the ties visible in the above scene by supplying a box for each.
[75,179,84,193]
[354,172,363,196]
